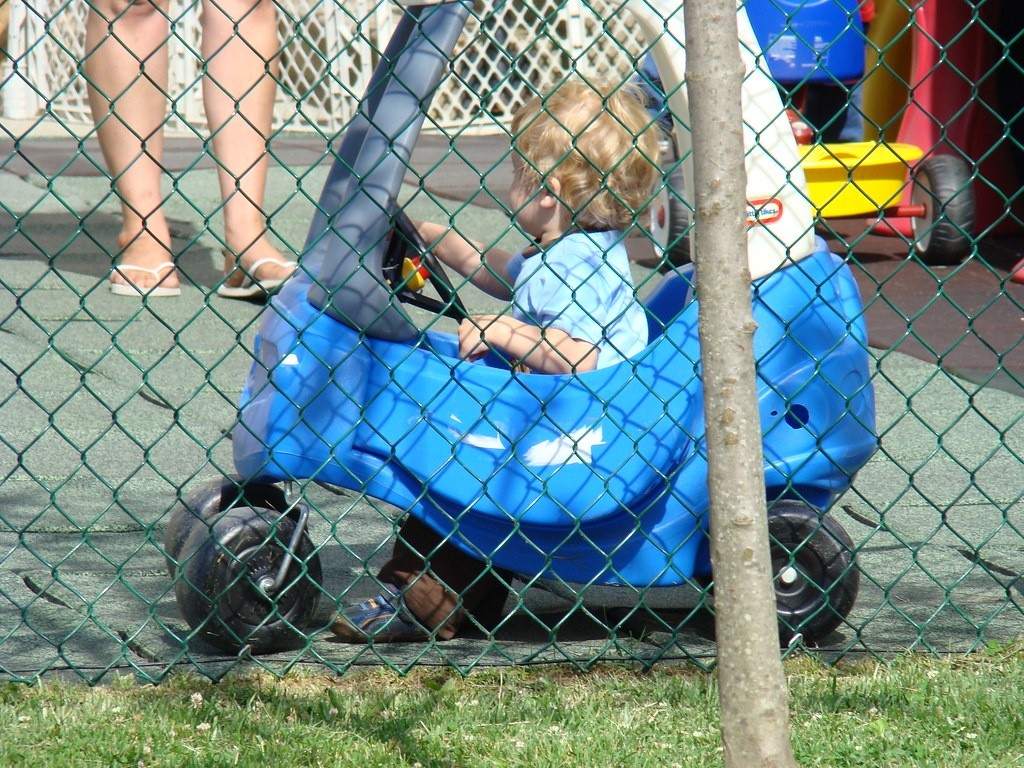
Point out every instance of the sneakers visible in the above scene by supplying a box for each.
[330,584,430,641]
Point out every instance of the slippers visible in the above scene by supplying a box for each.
[110,262,181,297]
[216,259,297,297]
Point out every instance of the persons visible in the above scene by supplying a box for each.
[330,82,660,635]
[85,0,297,296]
